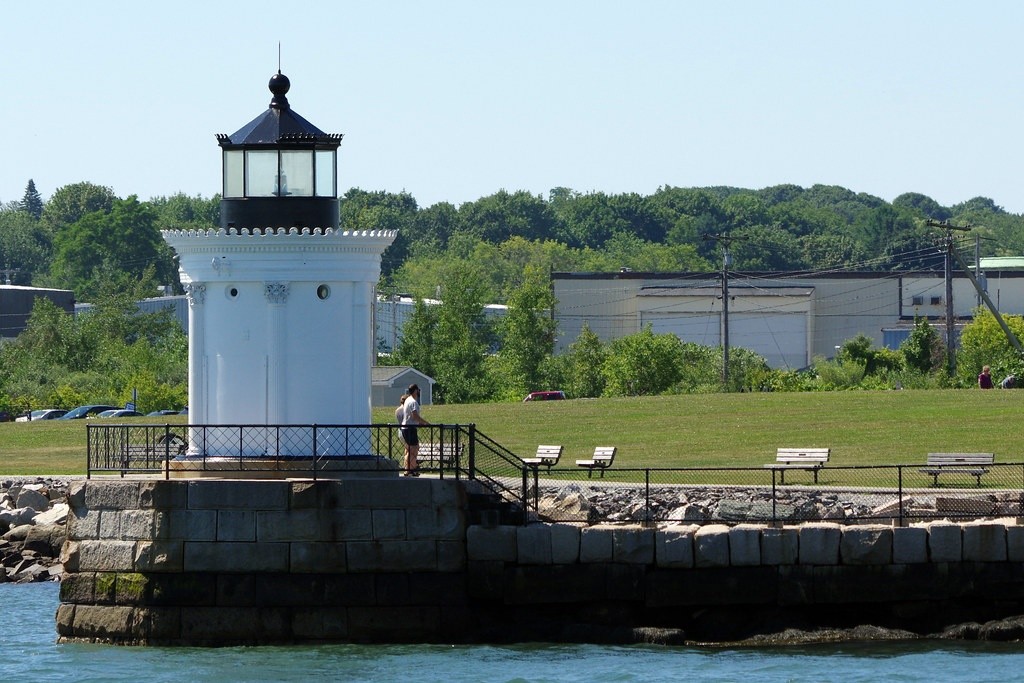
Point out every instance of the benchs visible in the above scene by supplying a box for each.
[576,447,617,477]
[764,448,830,484]
[416,443,464,470]
[112,444,180,473]
[919,453,994,487]
[521,445,563,475]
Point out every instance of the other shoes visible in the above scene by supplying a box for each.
[408,471,420,476]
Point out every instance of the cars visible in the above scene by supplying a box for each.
[57,405,120,419]
[14,409,71,422]
[97,410,144,417]
[147,410,180,416]
[523,391,565,401]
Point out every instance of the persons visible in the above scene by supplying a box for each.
[978,366,995,390]
[400,384,431,477]
[395,395,412,477]
[1001,375,1016,389]
